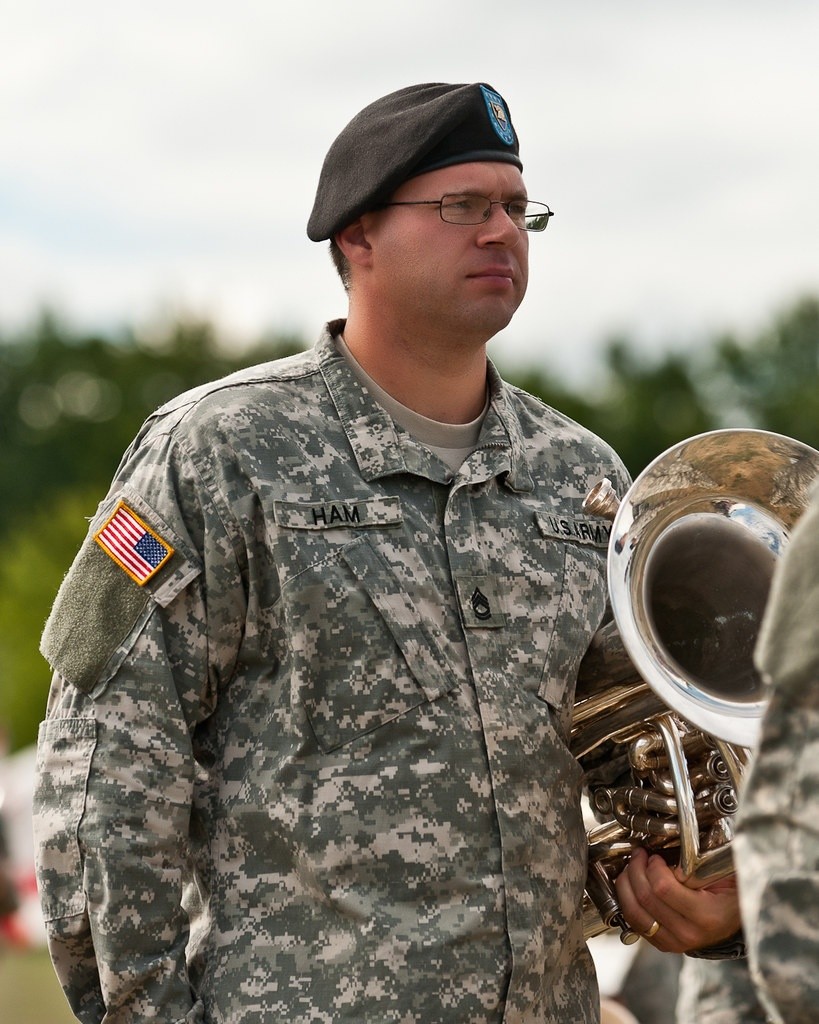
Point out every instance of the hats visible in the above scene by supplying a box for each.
[306,82,523,243]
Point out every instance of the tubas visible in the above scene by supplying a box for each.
[564,425,819,949]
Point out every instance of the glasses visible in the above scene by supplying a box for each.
[382,192,555,232]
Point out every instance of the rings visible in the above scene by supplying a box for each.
[644,921,659,938]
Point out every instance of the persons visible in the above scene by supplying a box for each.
[31,82,749,1024]
[731,472,819,1024]
